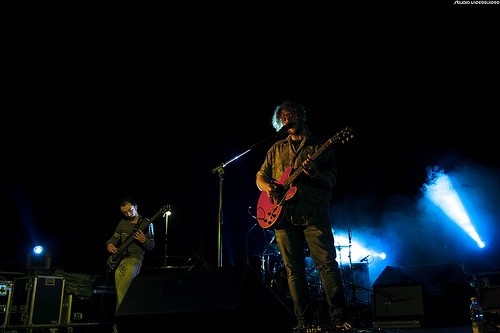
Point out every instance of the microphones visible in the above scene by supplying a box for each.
[275,123,294,137]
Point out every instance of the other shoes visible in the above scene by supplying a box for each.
[293,320,310,330]
[335,322,346,330]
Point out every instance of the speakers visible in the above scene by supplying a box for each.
[115,263,298,333]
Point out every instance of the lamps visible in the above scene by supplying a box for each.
[24,241,51,275]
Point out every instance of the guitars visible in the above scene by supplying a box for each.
[104,203,171,274]
[256,125,356,230]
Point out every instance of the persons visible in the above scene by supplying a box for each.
[105,199,155,310]
[256,101,354,333]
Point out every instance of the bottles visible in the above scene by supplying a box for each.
[470,297,487,333]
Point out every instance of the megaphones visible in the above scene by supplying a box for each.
[372,282,424,321]
